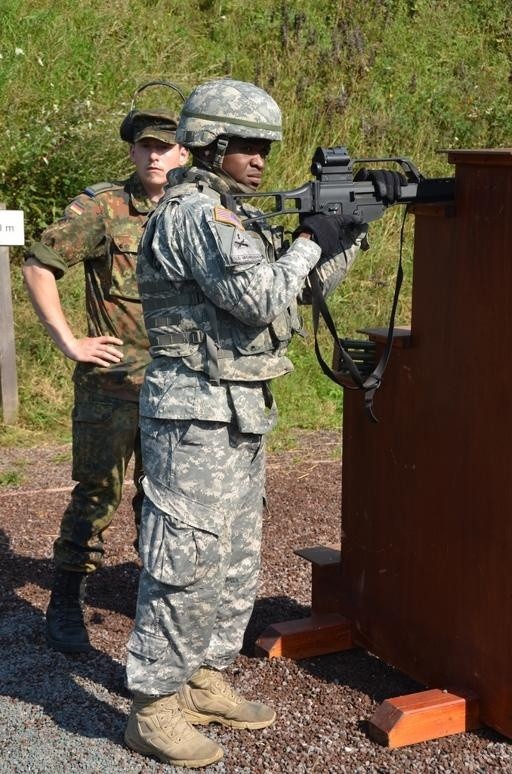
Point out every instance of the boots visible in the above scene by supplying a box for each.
[45,573,91,653]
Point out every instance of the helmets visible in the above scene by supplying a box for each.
[175,80,282,148]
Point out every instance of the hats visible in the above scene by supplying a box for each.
[120,108,180,145]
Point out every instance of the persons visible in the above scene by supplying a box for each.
[123,79,408,767]
[20,109,189,654]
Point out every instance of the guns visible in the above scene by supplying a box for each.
[219,146,454,233]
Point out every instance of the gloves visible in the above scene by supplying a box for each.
[353,168,407,205]
[292,211,361,259]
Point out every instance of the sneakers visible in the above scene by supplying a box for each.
[124,665,276,768]
